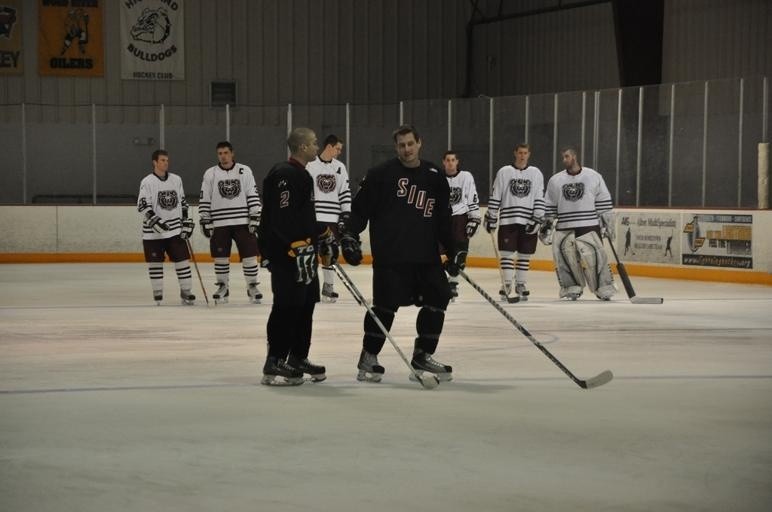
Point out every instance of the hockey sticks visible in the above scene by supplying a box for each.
[458,266,614,390]
[328,260,442,389]
[489,228,521,304]
[187,236,221,306]
[605,234,664,306]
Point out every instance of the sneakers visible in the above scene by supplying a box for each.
[515,284,529,295]
[213,284,228,298]
[287,359,326,374]
[322,283,339,298]
[247,285,263,299]
[181,290,195,300]
[263,357,303,378]
[412,351,452,373]
[357,348,384,373]
[449,282,459,297]
[153,290,163,300]
[499,284,511,295]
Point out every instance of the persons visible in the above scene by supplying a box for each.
[341,123,469,385]
[437,150,483,302]
[305,134,352,304]
[137,149,195,307]
[197,140,264,304]
[257,126,327,385]
[538,147,617,302]
[483,143,545,304]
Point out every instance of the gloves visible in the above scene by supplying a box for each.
[180,218,195,240]
[146,215,169,234]
[539,218,555,246]
[317,225,339,266]
[199,219,214,237]
[338,211,351,231]
[601,215,618,240]
[444,244,468,277]
[340,231,362,266]
[466,217,482,239]
[288,237,316,285]
[248,215,261,235]
[526,216,543,235]
[484,215,497,233]
